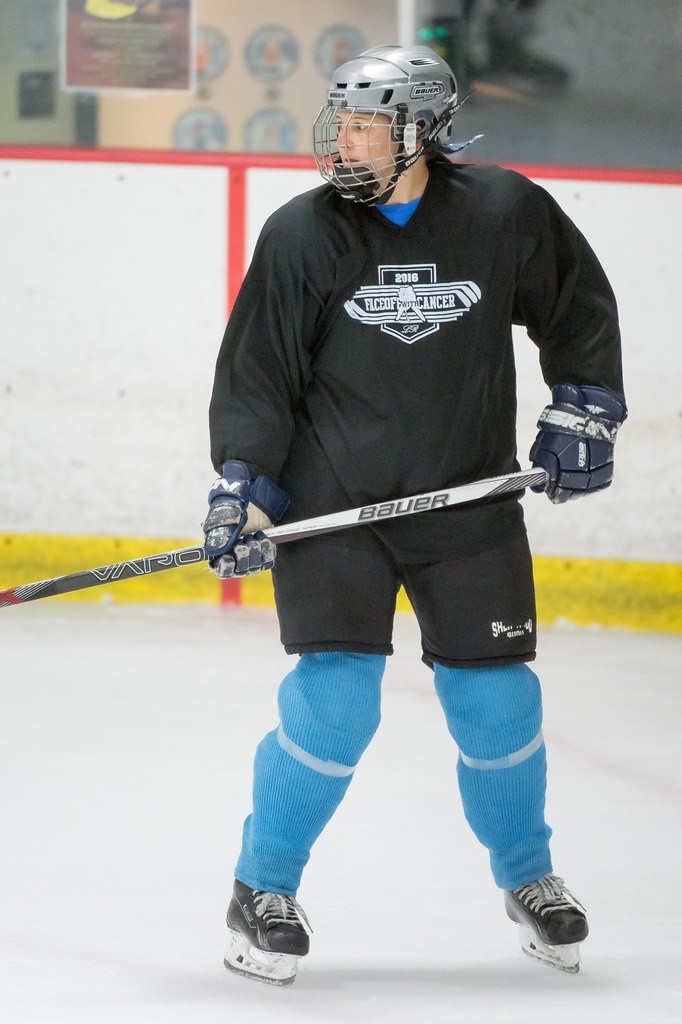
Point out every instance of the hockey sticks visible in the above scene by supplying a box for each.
[0,467,554,607]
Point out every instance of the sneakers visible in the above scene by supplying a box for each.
[504,877,587,974]
[224,878,311,987]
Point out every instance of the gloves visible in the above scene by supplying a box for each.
[530,381,627,504]
[207,460,290,578]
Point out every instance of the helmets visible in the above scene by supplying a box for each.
[313,44,460,204]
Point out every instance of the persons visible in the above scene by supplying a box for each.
[203,48,628,983]
